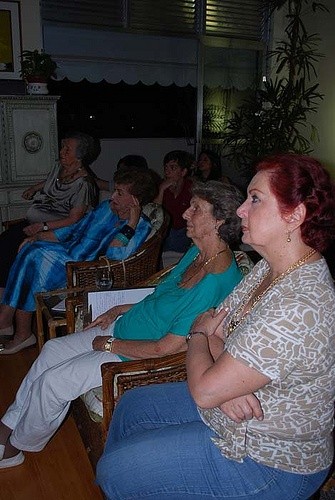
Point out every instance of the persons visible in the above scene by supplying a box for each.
[95,153,334,500]
[0,167,152,354]
[117,150,232,268]
[0,181,243,469]
[0,128,101,302]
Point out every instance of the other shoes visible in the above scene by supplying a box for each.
[0,333,36,354]
[0,325,13,335]
[0,444,25,469]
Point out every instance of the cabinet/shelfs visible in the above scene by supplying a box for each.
[0,95,61,231]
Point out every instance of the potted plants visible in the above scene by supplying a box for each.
[17,49,57,84]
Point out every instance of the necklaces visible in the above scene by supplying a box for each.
[180,249,227,283]
[227,247,318,330]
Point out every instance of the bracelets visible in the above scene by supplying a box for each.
[104,337,117,352]
[115,224,134,244]
[186,332,205,344]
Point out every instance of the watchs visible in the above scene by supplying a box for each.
[42,222,48,231]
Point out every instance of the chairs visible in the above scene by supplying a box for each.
[2,202,255,446]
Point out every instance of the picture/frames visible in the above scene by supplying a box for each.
[0,0,24,81]
